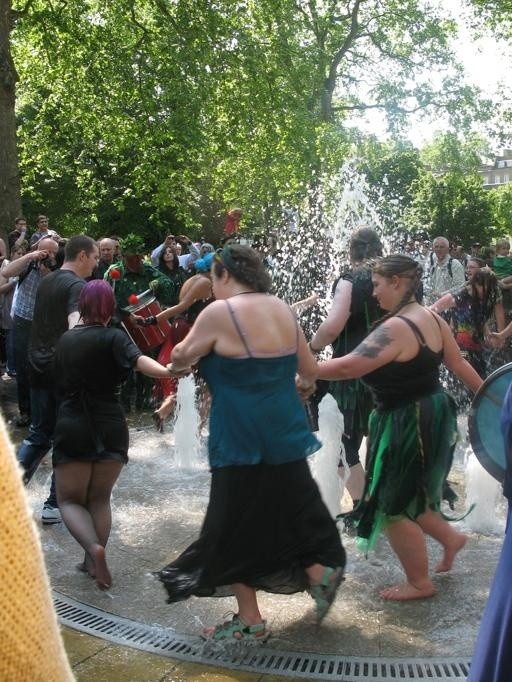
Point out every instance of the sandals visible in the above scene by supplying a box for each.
[309,567,343,625]
[200,615,270,644]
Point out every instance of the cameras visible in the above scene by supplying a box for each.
[40,255,51,266]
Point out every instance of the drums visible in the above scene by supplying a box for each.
[468,362,511,484]
[119,295,171,351]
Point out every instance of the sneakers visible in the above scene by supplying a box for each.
[17,413,30,427]
[42,505,63,524]
